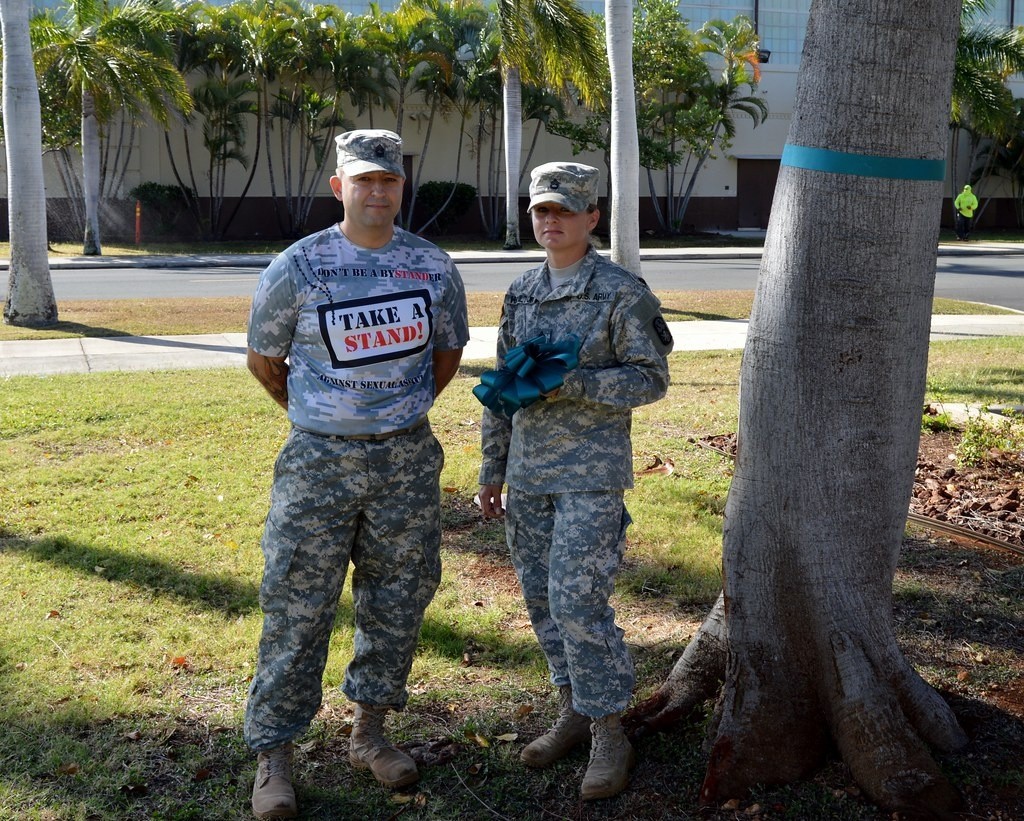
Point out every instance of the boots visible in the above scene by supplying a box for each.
[521,683,591,768]
[581,711,631,801]
[350,704,418,788]
[251,744,295,817]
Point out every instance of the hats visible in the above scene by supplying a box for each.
[335,129,405,177]
[527,161,599,214]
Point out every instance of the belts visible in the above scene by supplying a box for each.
[293,416,428,441]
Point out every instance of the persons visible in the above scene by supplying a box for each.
[478,162,675,798]
[953,184,979,241]
[243,129,470,817]
